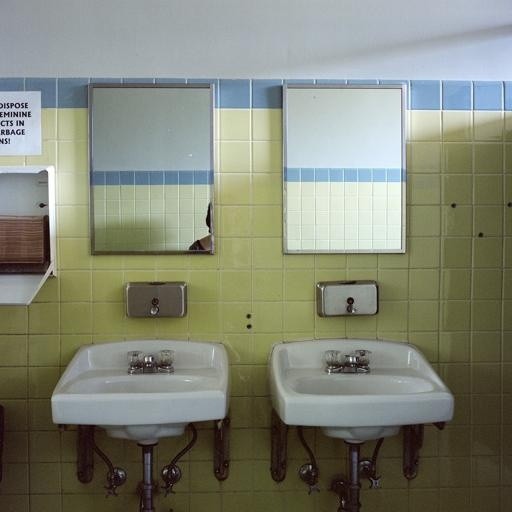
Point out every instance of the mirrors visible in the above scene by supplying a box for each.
[281,83,407,254]
[0,165,56,307]
[88,82,215,257]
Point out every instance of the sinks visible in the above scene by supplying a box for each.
[51,340,228,426]
[264,339,454,427]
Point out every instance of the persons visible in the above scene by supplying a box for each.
[189,202,212,250]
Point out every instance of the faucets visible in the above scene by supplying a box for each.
[343,355,357,373]
[144,355,158,374]
